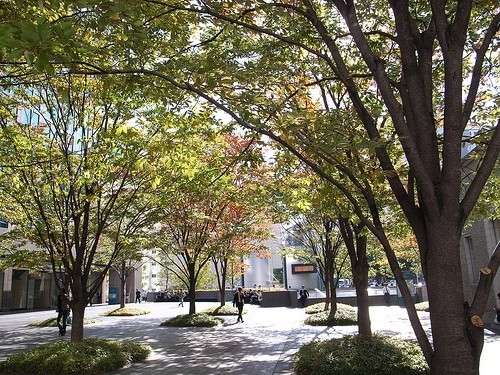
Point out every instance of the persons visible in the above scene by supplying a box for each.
[56,288,70,336]
[243,289,263,306]
[496,293,500,324]
[178,289,184,307]
[383,283,392,304]
[135,289,141,303]
[300,285,309,307]
[232,287,246,323]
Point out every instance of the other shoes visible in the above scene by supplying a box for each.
[242,321,244,323]
[237,319,240,322]
